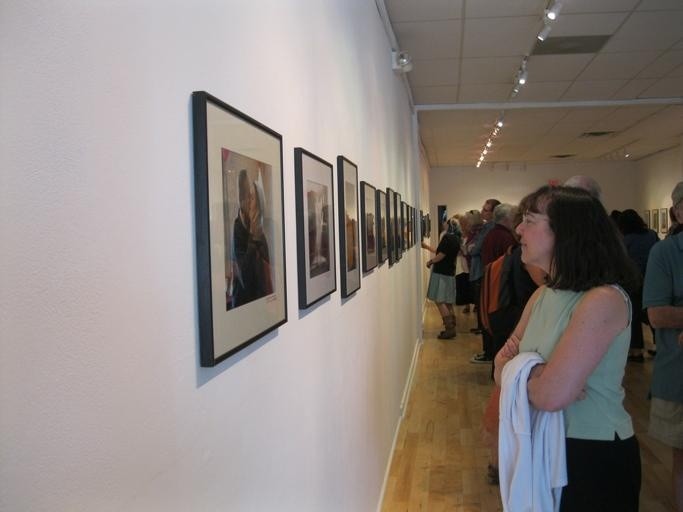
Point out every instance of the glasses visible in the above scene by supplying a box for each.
[674,198,683,208]
[520,214,548,225]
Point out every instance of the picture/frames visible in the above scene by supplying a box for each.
[338,156,360,299]
[361,181,431,272]
[193,91,286,367]
[294,147,336,309]
[643,208,668,233]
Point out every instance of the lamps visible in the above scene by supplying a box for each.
[622,146,631,158]
[519,67,528,85]
[496,117,506,127]
[392,49,415,74]
[548,1,563,20]
[536,25,553,43]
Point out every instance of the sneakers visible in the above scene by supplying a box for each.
[471,328,481,334]
[629,353,645,361]
[486,462,499,483]
[470,352,493,363]
[647,350,656,357]
[463,306,477,313]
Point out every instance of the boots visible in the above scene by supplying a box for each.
[437,315,454,339]
[440,315,456,336]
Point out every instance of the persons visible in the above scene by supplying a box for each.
[422,209,486,339]
[494,185,641,512]
[231,169,273,305]
[472,195,547,486]
[610,207,683,364]
[642,182,682,512]
